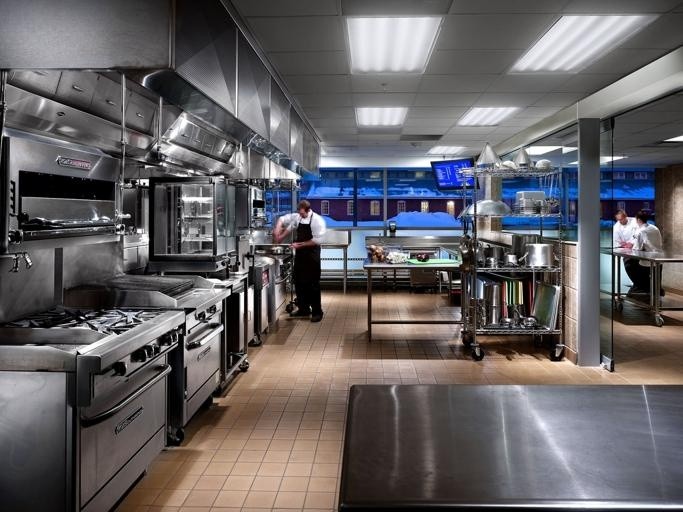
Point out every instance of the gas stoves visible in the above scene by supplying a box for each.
[1,303,186,408]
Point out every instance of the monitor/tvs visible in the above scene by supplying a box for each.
[430,156,481,192]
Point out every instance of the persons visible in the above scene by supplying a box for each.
[612,206,652,304]
[272,210,302,241]
[625,204,665,308]
[273,199,327,323]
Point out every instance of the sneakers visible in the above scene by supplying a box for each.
[291,307,311,316]
[310,313,323,322]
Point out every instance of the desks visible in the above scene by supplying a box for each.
[609,247,682,327]
[363,259,464,343]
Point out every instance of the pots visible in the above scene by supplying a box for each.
[270,242,304,255]
[459,234,560,329]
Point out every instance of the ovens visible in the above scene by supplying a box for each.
[71,354,168,512]
[182,300,224,433]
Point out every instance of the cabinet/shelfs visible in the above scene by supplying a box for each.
[460,165,562,361]
